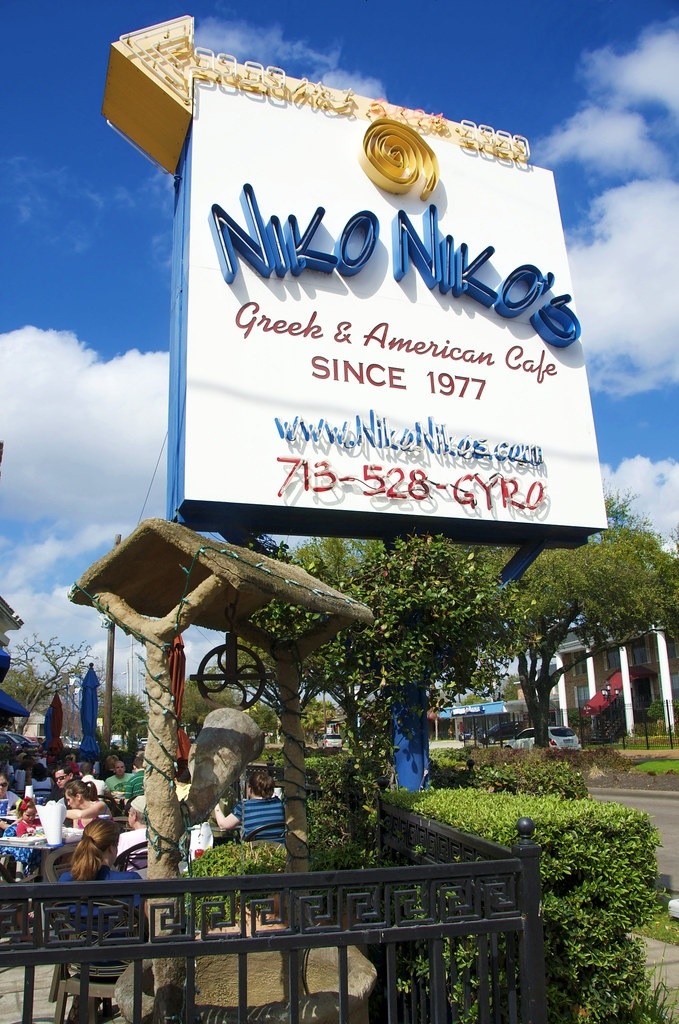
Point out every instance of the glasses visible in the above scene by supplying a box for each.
[0,783,8,787]
[55,773,67,782]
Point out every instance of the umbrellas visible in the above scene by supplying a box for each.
[166,634,192,778]
[0,689,30,717]
[41,706,52,750]
[48,689,63,748]
[77,663,101,762]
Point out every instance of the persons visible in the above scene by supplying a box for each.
[214,772,284,843]
[448,724,454,740]
[0,738,214,942]
[54,819,147,985]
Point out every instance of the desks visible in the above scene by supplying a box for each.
[0,844,64,883]
[209,828,240,844]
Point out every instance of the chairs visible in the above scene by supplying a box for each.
[243,822,285,842]
[0,864,43,937]
[34,788,52,798]
[98,795,139,831]
[45,842,147,1024]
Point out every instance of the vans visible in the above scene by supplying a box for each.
[316,734,342,748]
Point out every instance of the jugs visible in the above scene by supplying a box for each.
[15,770,26,791]
[35,800,68,845]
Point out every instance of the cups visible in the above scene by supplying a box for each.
[98,814,110,819]
[35,827,43,836]
[36,797,44,804]
[0,799,9,816]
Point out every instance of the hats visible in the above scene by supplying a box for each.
[131,795,145,813]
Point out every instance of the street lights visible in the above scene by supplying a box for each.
[601,683,620,739]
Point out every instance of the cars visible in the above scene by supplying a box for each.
[59,735,82,747]
[140,738,148,744]
[0,731,36,750]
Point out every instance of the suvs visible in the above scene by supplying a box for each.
[480,722,523,744]
[503,726,582,750]
